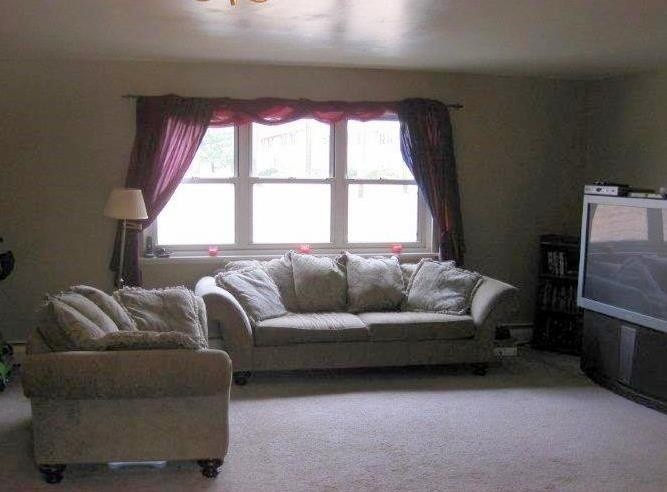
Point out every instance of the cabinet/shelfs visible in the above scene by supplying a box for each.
[580,311,667,414]
[531,233,584,356]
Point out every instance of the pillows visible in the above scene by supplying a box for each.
[214,266,288,321]
[341,250,406,314]
[290,250,348,312]
[226,250,297,313]
[36,284,208,351]
[401,258,484,314]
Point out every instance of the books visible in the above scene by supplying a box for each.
[539,251,585,350]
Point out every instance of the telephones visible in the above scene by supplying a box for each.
[145,236,153,258]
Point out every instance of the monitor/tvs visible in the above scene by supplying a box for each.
[576,195,667,333]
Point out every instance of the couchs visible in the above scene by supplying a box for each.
[18,286,235,484]
[195,250,523,386]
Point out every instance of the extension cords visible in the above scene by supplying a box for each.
[493,347,518,356]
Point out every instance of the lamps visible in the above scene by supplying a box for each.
[102,187,149,290]
